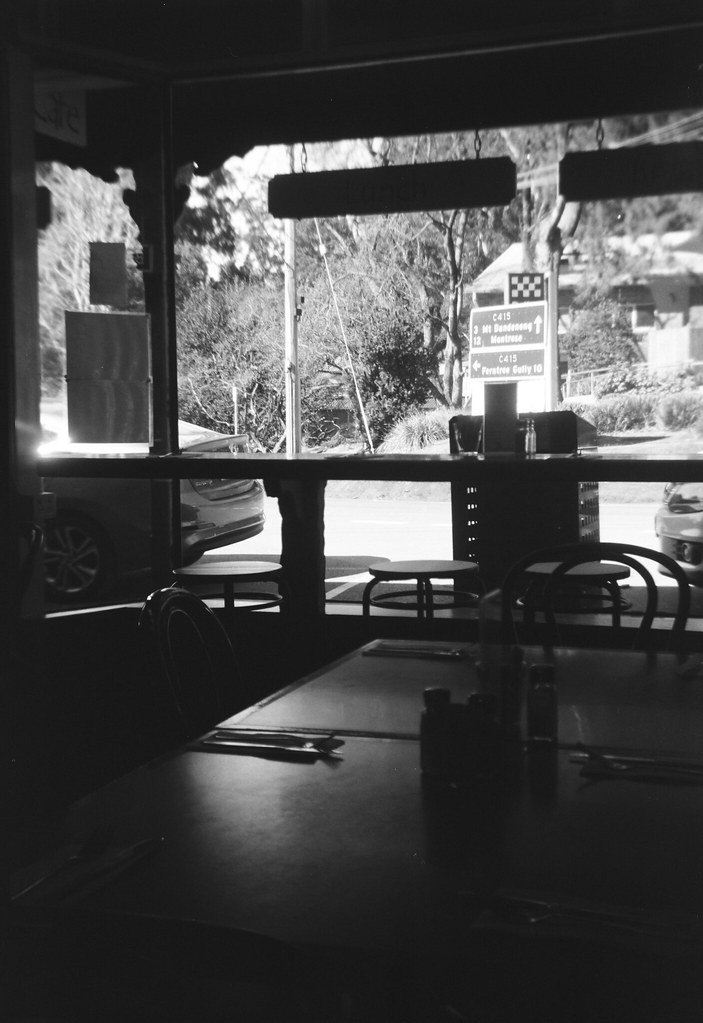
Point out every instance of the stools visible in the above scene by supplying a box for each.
[512,562,630,643]
[171,563,283,638]
[363,560,487,643]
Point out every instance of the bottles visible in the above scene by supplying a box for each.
[514,418,536,455]
[527,663,559,742]
[420,686,450,774]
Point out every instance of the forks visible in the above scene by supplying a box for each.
[10,823,119,903]
[576,741,703,775]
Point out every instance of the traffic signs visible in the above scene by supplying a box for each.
[468,300,547,348]
[467,347,545,382]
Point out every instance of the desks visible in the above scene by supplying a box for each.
[0,638,703,1023]
[32,453,703,667]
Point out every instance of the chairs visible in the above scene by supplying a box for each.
[139,586,242,746]
[501,543,690,652]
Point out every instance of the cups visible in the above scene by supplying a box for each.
[453,422,482,455]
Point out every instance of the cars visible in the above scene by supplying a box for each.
[35,404,266,607]
[652,482,703,585]
